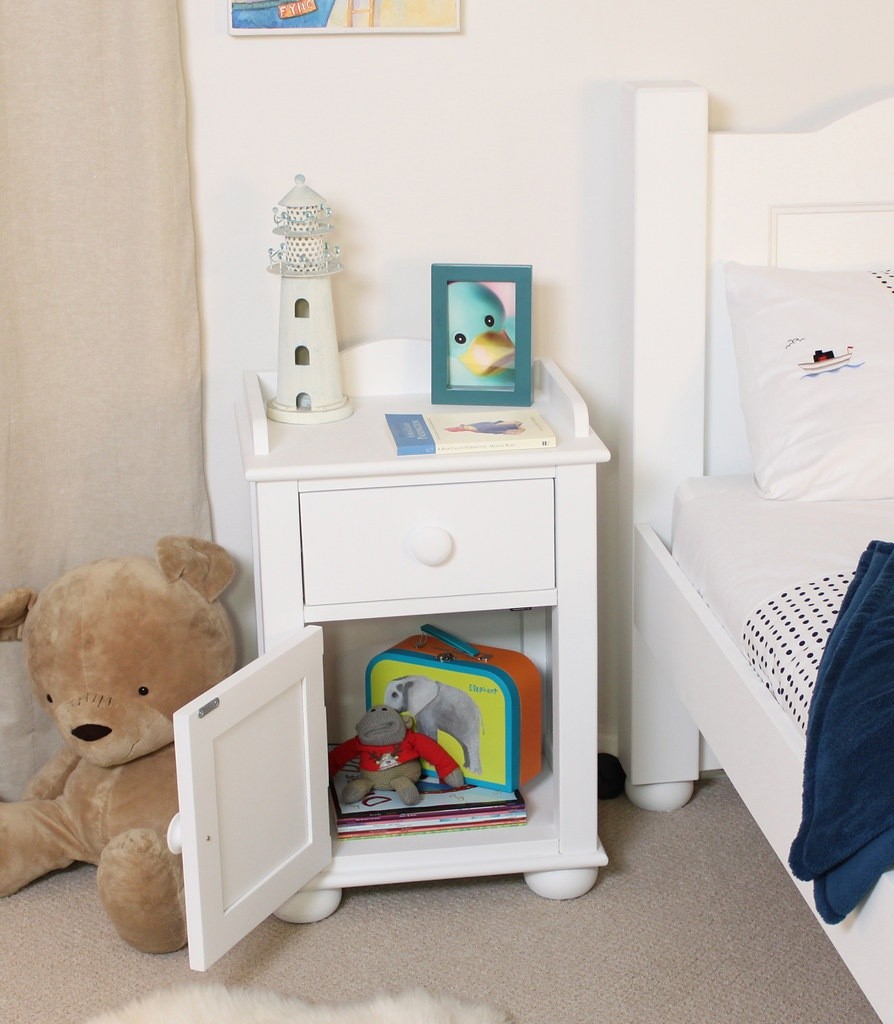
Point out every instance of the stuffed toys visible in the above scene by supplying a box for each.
[328,705,463,805]
[0,533,237,954]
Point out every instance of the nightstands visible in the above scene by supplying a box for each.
[172,340,610,958]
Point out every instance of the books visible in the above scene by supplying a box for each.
[328,744,527,842]
[382,410,557,455]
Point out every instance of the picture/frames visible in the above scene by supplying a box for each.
[430,265,531,405]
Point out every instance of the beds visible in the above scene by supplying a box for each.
[620,83,894,1024]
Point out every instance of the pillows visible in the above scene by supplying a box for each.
[721,268,894,504]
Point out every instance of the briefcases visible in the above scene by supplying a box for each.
[365,623,542,791]
